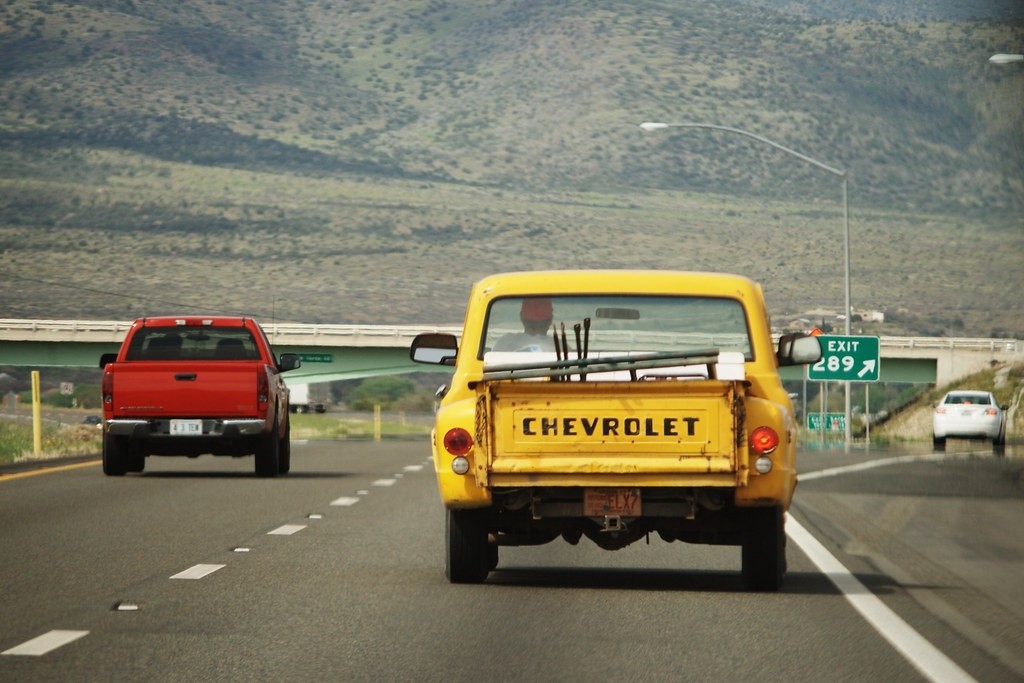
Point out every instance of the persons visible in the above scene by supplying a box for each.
[492,291,582,354]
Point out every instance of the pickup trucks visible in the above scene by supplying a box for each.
[100,317,301,479]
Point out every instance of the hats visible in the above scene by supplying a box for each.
[521,297,552,321]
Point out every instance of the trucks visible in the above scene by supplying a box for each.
[287,382,329,415]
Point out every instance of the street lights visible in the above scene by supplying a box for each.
[641,121,852,456]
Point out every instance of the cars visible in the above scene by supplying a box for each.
[933,390,1010,454]
[410,270,823,593]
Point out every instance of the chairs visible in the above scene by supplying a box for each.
[217,338,247,358]
